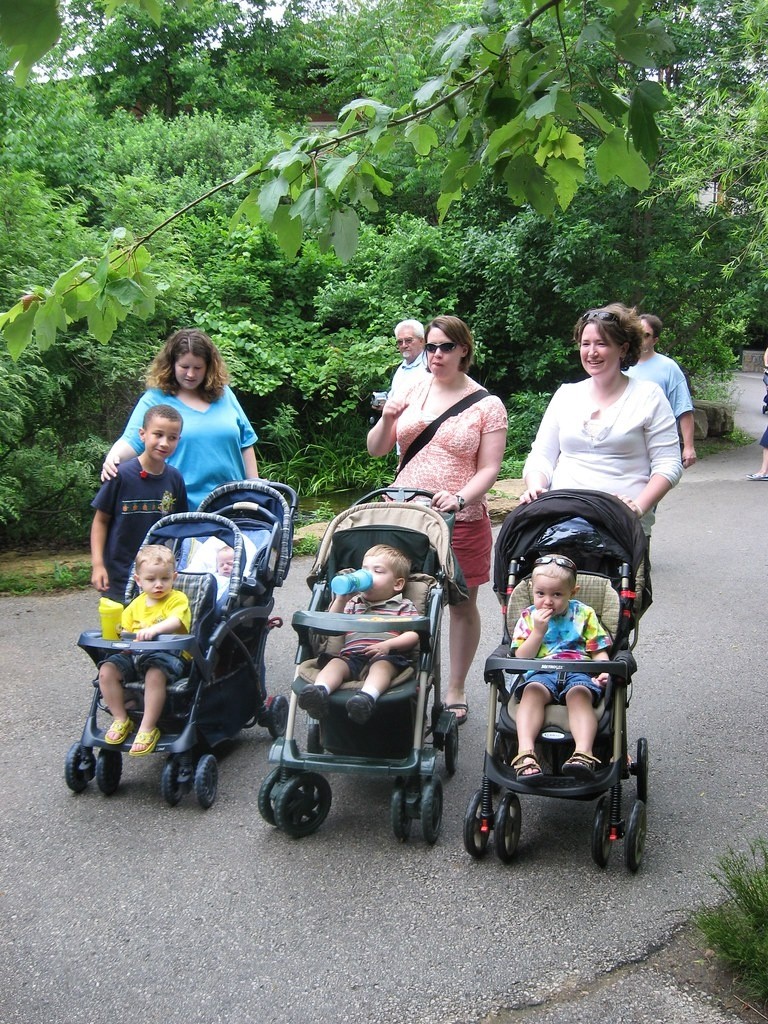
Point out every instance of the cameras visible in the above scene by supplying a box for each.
[371,390,387,406]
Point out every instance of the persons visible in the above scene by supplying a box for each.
[746,348,768,481]
[212,545,234,601]
[368,316,508,726]
[91,404,188,610]
[520,304,684,766]
[621,314,697,515]
[298,544,418,723]
[98,545,194,756]
[373,320,427,456]
[101,329,259,511]
[510,555,613,781]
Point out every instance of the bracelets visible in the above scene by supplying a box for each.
[634,503,643,516]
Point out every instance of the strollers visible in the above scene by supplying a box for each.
[256,487,467,846]
[65,478,288,808]
[461,488,654,875]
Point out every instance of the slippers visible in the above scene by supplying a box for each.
[105,715,135,744]
[128,727,161,757]
[444,700,470,726]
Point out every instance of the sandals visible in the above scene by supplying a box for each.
[297,683,330,720]
[345,690,375,726]
[510,749,543,781]
[561,752,602,777]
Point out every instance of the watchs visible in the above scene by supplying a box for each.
[456,495,465,511]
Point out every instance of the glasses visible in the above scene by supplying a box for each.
[580,312,619,322]
[424,341,459,353]
[395,338,414,345]
[533,556,577,576]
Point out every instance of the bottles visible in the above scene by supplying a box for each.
[98,598,123,641]
[331,569,373,595]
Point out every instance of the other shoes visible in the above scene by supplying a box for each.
[746,473,768,481]
